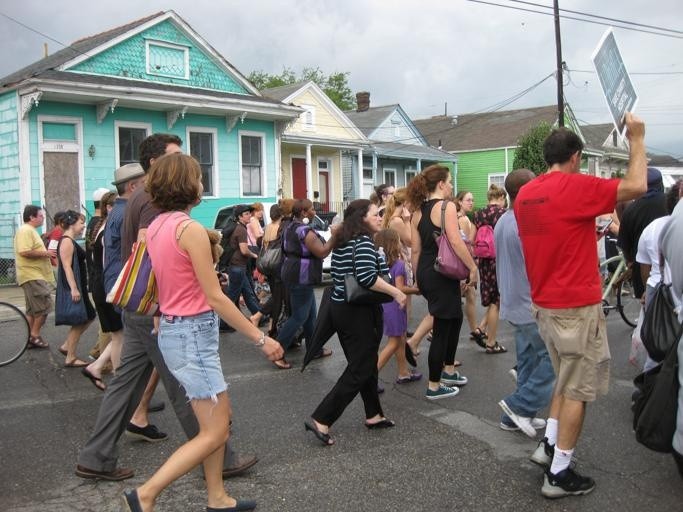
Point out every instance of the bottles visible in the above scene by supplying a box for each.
[459,229,466,241]
[376,247,385,262]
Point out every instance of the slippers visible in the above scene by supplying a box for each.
[57,346,86,368]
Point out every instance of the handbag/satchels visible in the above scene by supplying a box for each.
[434,200,471,280]
[343,242,395,306]
[106,241,162,317]
[257,237,286,275]
[632,326,682,454]
[640,256,683,365]
[54,278,89,327]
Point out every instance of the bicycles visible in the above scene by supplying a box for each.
[598,234,649,331]
[0,300,35,369]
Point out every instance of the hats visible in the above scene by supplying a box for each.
[646,167,665,198]
[111,163,147,185]
[93,188,108,202]
[234,205,254,217]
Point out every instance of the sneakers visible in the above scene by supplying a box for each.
[529,436,555,468]
[121,489,143,512]
[509,367,519,384]
[405,340,419,368]
[247,312,332,371]
[397,372,423,385]
[423,384,460,401]
[124,421,168,445]
[497,398,547,438]
[441,373,469,387]
[540,466,595,499]
[203,498,258,511]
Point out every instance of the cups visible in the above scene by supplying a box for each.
[47,248,56,260]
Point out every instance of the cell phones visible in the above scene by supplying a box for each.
[596,219,613,233]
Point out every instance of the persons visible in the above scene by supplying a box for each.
[403,163,468,401]
[512,108,650,497]
[100,159,171,445]
[71,134,258,482]
[119,152,287,512]
[619,167,667,299]
[490,167,557,442]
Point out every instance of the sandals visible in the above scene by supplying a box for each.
[83,366,105,394]
[28,334,50,348]
[485,341,509,355]
[304,419,336,446]
[470,327,488,350]
[364,418,397,430]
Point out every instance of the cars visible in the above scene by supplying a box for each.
[242,205,339,286]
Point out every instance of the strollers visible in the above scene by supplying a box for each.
[246,245,277,327]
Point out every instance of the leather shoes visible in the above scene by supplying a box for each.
[201,454,259,480]
[75,464,134,483]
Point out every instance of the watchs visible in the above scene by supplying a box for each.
[251,332,266,349]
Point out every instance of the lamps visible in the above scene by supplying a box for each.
[87,144,96,161]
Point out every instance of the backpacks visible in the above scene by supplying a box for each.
[475,210,498,262]
[221,222,238,267]
